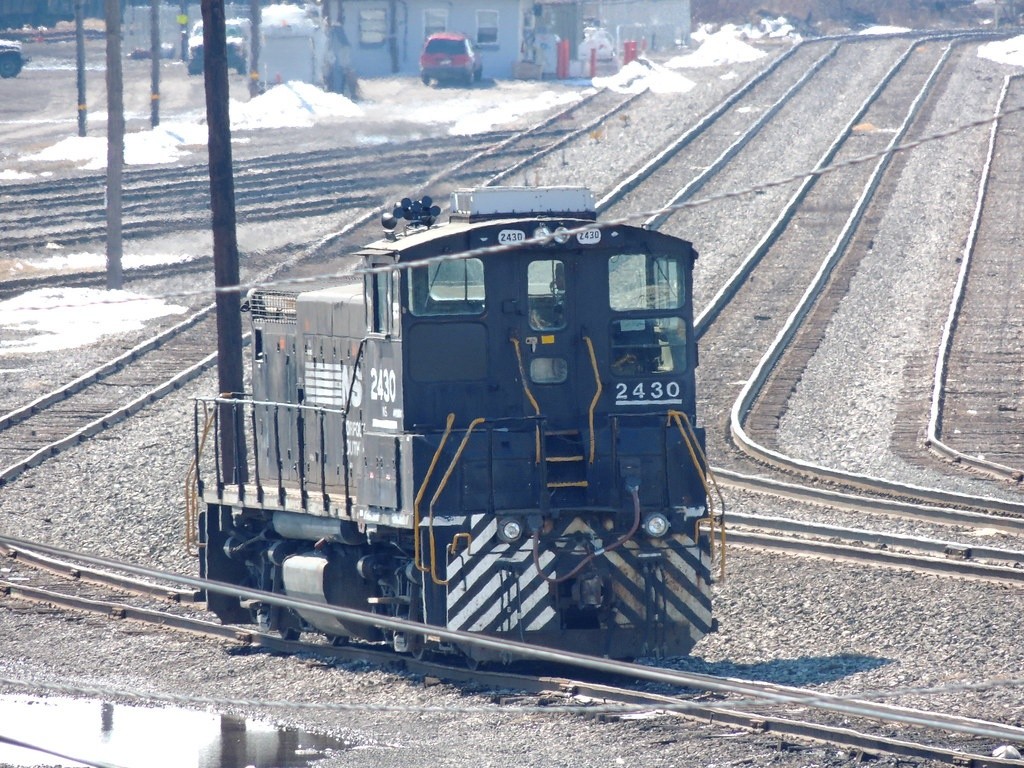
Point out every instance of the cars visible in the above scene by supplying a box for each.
[420,32,483,86]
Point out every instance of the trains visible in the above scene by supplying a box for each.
[184,182,727,668]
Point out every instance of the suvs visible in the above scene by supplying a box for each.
[181,17,253,77]
